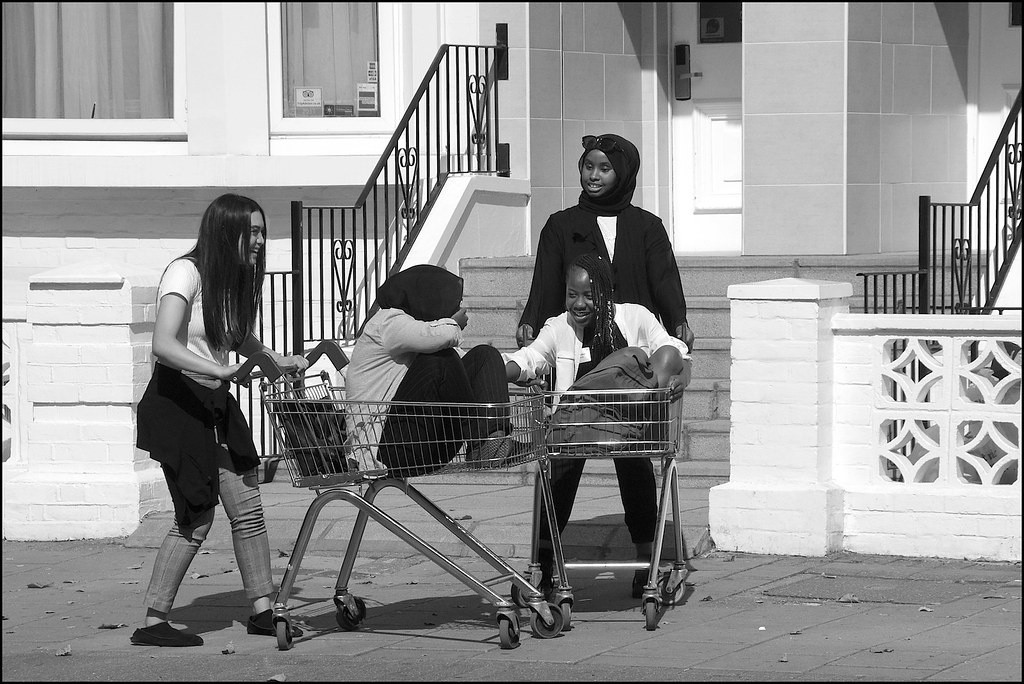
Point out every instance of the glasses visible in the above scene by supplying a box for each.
[580,135,629,160]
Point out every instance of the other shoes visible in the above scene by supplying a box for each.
[503,438,534,463]
[247,615,302,637]
[130,629,203,647]
[466,431,510,468]
[632,571,648,598]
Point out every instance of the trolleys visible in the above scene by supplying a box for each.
[519,322,688,632]
[230,338,563,650]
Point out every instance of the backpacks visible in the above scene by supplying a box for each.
[545,353,659,453]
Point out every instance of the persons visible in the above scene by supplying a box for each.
[344,264,548,480]
[515,134,695,600]
[501,254,694,450]
[130,193,309,647]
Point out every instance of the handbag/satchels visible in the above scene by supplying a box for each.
[273,395,347,475]
